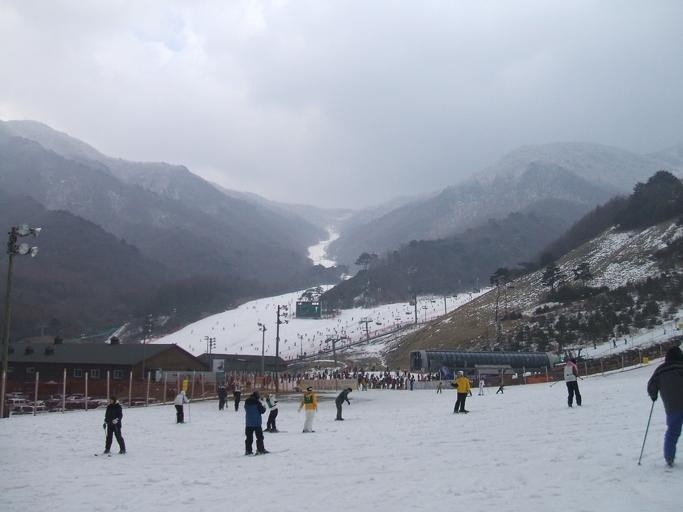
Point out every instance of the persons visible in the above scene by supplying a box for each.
[478,376,484,395]
[173,391,189,424]
[646,345,682,467]
[232,381,240,411]
[496,384,504,393]
[435,381,443,394]
[244,390,267,454]
[563,358,583,407]
[452,370,471,413]
[264,392,278,431]
[224,367,415,391]
[101,395,126,454]
[217,379,227,410]
[334,386,351,420]
[296,386,315,432]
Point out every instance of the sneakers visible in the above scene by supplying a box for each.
[246,452,253,455]
[119,451,125,454]
[257,450,269,454]
[264,429,277,432]
[105,450,110,454]
[455,410,469,413]
[666,456,674,465]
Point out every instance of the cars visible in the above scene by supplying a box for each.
[5,391,156,414]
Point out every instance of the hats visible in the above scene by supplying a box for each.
[110,396,116,400]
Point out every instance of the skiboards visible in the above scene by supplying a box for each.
[246,448,290,457]
[263,429,288,432]
[94,449,125,456]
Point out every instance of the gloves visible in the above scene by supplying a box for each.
[112,418,119,424]
[103,424,106,429]
[652,396,657,401]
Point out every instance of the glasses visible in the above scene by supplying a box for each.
[308,390,312,391]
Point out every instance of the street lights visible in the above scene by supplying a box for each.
[324,337,340,368]
[0,225,42,419]
[261,325,267,377]
[207,337,216,371]
[357,317,373,343]
[140,313,152,380]
[273,304,289,392]
